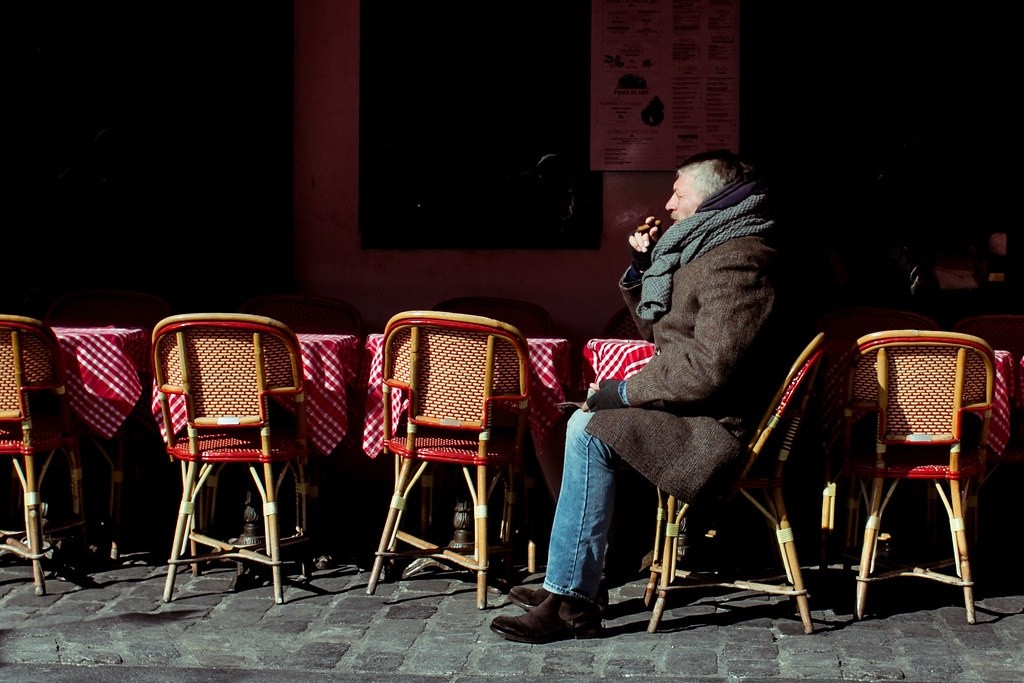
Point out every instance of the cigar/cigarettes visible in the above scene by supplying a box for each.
[636,219,664,233]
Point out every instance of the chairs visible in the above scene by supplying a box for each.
[641,328,827,636]
[812,308,943,342]
[364,310,534,611]
[234,294,364,338]
[150,311,311,605]
[432,293,560,339]
[0,314,90,598]
[817,328,997,626]
[951,315,1024,365]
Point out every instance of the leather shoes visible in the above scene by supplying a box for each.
[508,586,609,611]
[490,592,600,644]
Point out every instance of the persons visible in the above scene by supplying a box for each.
[490,147,806,645]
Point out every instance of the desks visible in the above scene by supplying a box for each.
[45,324,1016,569]
